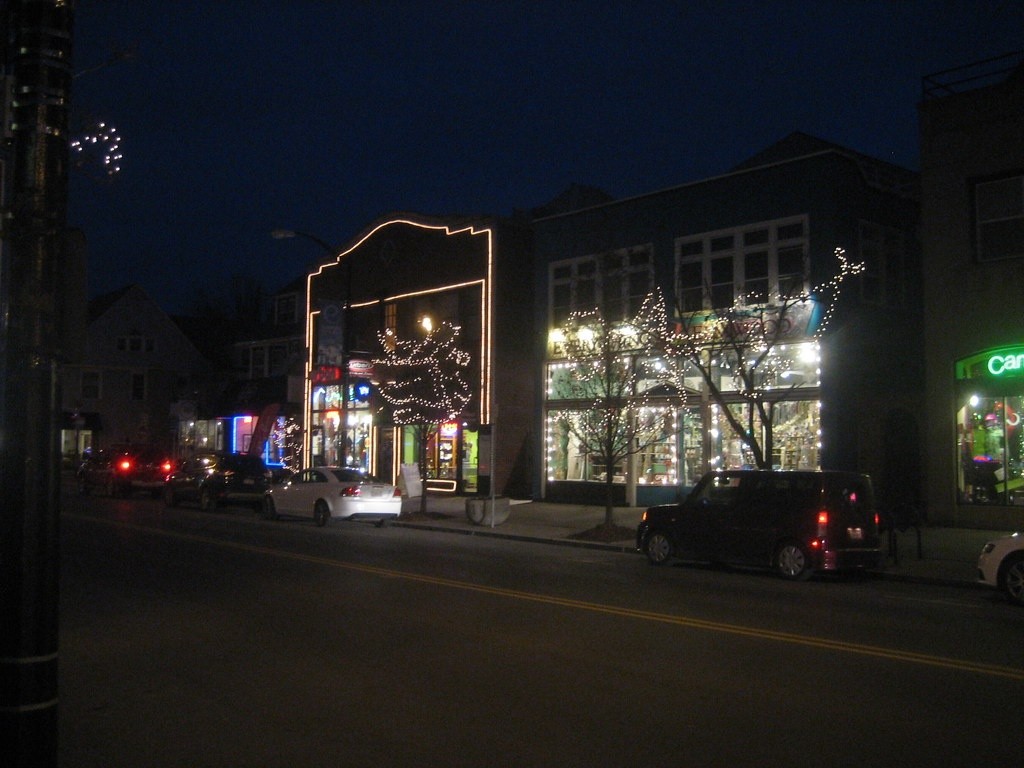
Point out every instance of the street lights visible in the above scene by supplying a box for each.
[271,229,354,468]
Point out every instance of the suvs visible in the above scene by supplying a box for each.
[636,469,884,582]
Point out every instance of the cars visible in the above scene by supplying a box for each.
[259,466,403,529]
[163,451,274,513]
[977,529,1024,606]
[77,443,178,498]
